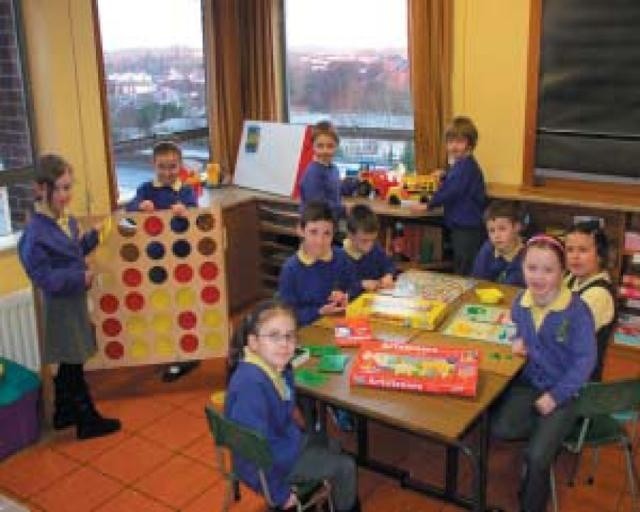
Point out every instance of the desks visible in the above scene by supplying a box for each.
[277,268,544,511]
[421,269,532,381]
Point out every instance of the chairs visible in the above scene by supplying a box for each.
[541,350,640,511]
[187,401,340,511]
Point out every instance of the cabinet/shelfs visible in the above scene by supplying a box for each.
[251,186,452,300]
[479,178,640,391]
[192,180,266,318]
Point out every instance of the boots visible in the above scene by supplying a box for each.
[52,374,120,440]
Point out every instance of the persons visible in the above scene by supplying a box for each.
[474,234,599,511]
[120,141,199,383]
[466,197,536,287]
[297,120,352,223]
[563,219,621,381]
[16,154,121,439]
[220,299,361,511]
[339,204,395,291]
[407,115,488,273]
[274,199,361,432]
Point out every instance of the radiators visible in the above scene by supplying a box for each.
[0,288,55,374]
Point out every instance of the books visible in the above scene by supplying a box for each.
[347,335,482,397]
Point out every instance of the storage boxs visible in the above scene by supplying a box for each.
[0,350,41,461]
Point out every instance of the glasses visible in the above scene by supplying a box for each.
[256,331,299,343]
